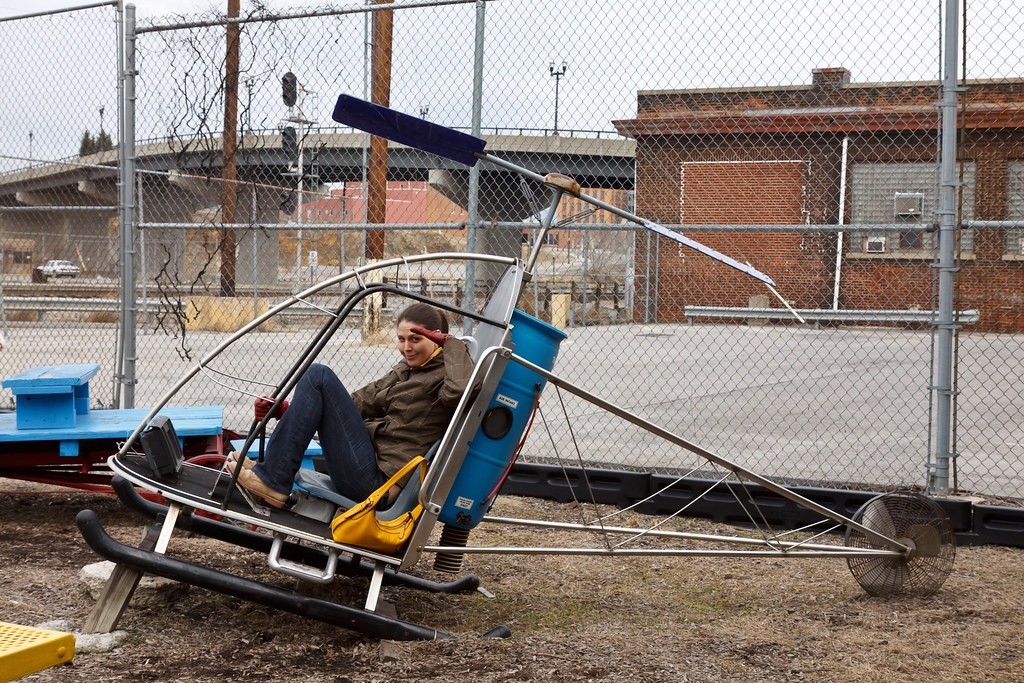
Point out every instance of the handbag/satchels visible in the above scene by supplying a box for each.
[331,456,427,555]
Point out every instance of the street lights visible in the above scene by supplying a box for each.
[549,61,568,136]
[28,129,34,169]
[419,103,429,120]
[244,75,254,136]
[97,104,105,152]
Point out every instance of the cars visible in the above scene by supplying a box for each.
[38,260,81,278]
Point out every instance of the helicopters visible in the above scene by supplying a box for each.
[69,94,958,657]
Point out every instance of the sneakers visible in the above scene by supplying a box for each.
[233,451,257,469]
[225,461,288,508]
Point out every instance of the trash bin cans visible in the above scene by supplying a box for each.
[749,295,770,325]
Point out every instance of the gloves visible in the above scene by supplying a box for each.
[254,395,288,420]
[410,328,452,348]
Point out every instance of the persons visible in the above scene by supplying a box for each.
[227,303,479,509]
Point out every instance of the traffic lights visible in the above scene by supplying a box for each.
[281,71,297,108]
[281,127,298,161]
